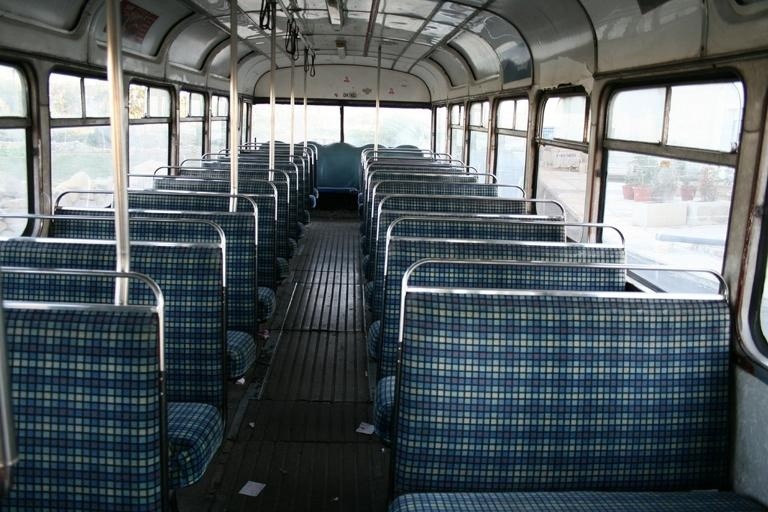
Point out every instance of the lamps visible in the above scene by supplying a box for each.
[334,40,347,59]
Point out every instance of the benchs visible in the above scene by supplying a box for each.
[0,141,768,512]
